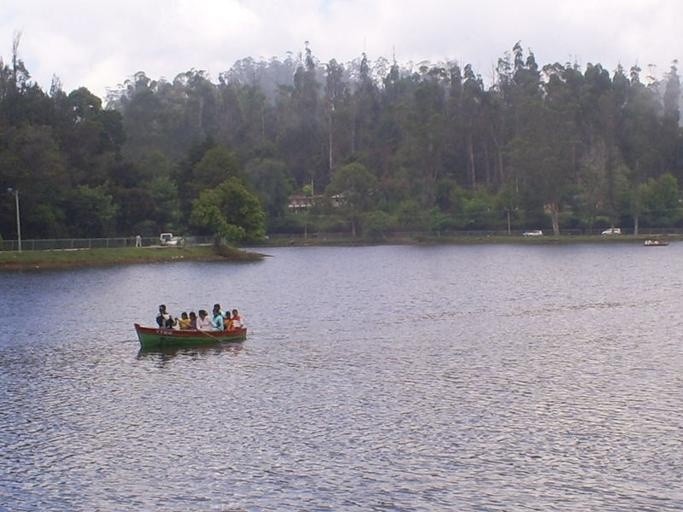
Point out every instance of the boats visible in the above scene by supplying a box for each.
[132,321,248,353]
[643,241,670,246]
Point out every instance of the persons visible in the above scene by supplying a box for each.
[136,234,142,247]
[156,304,243,331]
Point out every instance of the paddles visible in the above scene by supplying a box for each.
[174,318,223,343]
